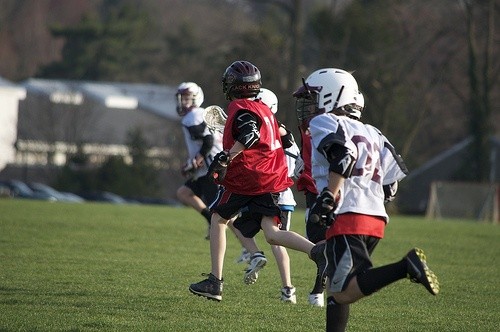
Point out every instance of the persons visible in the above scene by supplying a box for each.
[293,68,439,332]
[176,82,250,263]
[228,88,300,304]
[293,85,325,306]
[188,61,328,303]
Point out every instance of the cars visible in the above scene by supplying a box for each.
[0,178,182,206]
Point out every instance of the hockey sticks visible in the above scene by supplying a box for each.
[201,104,298,160]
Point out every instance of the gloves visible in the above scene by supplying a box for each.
[309,187,340,228]
[181,161,195,178]
[206,151,232,184]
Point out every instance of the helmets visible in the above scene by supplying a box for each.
[293,68,359,113]
[221,61,262,100]
[175,82,204,115]
[248,88,278,115]
[348,91,364,120]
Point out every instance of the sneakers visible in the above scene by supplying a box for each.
[405,247,440,295]
[188,272,224,301]
[238,249,249,262]
[310,239,329,282]
[310,291,324,307]
[280,286,296,304]
[244,251,267,285]
